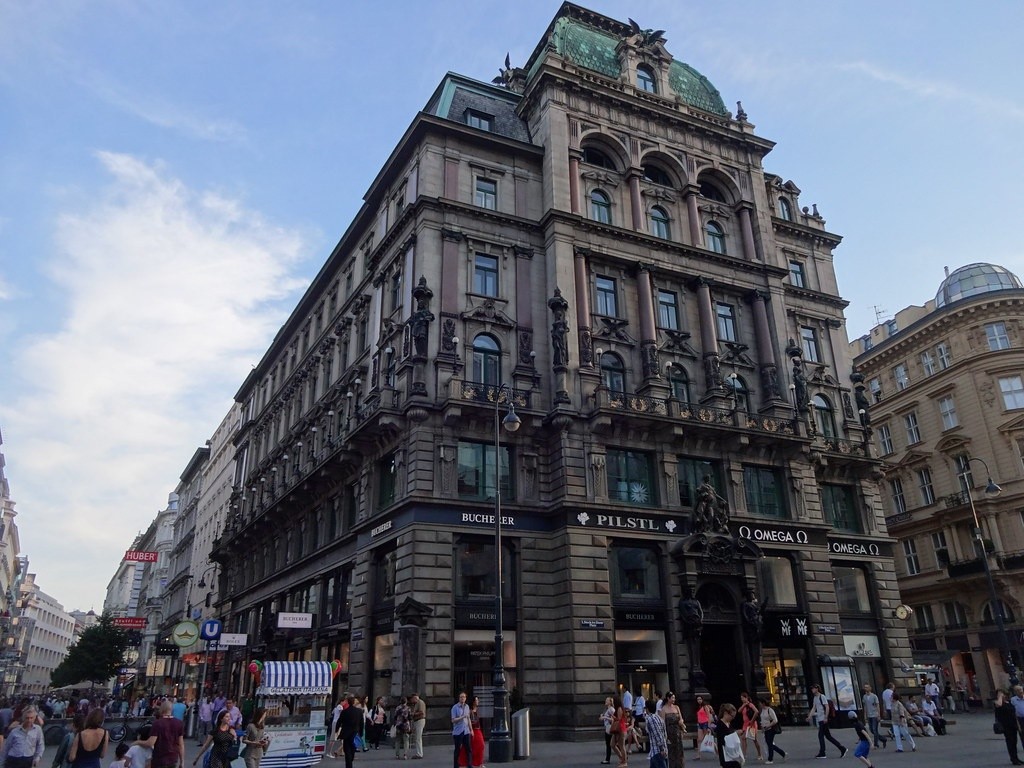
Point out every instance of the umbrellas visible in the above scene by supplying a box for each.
[51,680,109,692]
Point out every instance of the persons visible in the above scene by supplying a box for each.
[848,711,875,768]
[551,311,567,365]
[793,360,810,407]
[806,684,848,758]
[327,693,387,758]
[994,684,1024,766]
[335,697,365,768]
[598,684,788,768]
[862,678,969,752]
[409,693,426,759]
[393,697,413,759]
[694,473,728,533]
[409,301,435,357]
[855,386,870,425]
[0,690,270,768]
[451,691,485,768]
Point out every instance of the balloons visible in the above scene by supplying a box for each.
[331,659,341,672]
[249,659,262,672]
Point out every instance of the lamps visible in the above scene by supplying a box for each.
[384,347,392,385]
[215,477,265,542]
[294,441,303,477]
[282,455,289,491]
[789,383,797,415]
[731,373,740,409]
[327,411,334,446]
[858,408,867,438]
[530,351,538,388]
[271,467,278,501]
[452,337,459,375]
[310,426,317,462]
[596,348,604,385]
[665,361,674,397]
[354,379,362,417]
[346,392,353,426]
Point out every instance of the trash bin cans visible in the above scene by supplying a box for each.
[511,707,531,760]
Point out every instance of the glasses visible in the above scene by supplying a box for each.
[669,691,674,695]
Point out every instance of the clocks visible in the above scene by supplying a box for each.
[896,605,907,619]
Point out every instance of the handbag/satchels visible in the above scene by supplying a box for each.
[993,721,1005,734]
[229,729,241,761]
[605,716,613,734]
[819,694,835,719]
[609,719,619,734]
[700,732,714,752]
[390,725,396,738]
[746,725,755,740]
[770,720,782,734]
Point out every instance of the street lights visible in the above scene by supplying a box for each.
[196,566,225,700]
[143,609,165,716]
[486,382,523,765]
[962,457,1019,687]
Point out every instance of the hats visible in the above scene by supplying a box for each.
[848,710,857,719]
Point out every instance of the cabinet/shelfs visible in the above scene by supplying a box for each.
[773,675,810,714]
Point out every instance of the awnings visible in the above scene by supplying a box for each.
[257,660,333,707]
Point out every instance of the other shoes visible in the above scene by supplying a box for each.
[758,756,763,760]
[1012,761,1021,765]
[1019,760,1024,764]
[841,747,848,758]
[815,751,826,759]
[601,744,642,768]
[693,756,700,760]
[784,752,787,762]
[765,760,773,764]
[327,742,423,760]
[874,730,947,752]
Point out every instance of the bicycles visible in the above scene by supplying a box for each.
[108,713,153,743]
